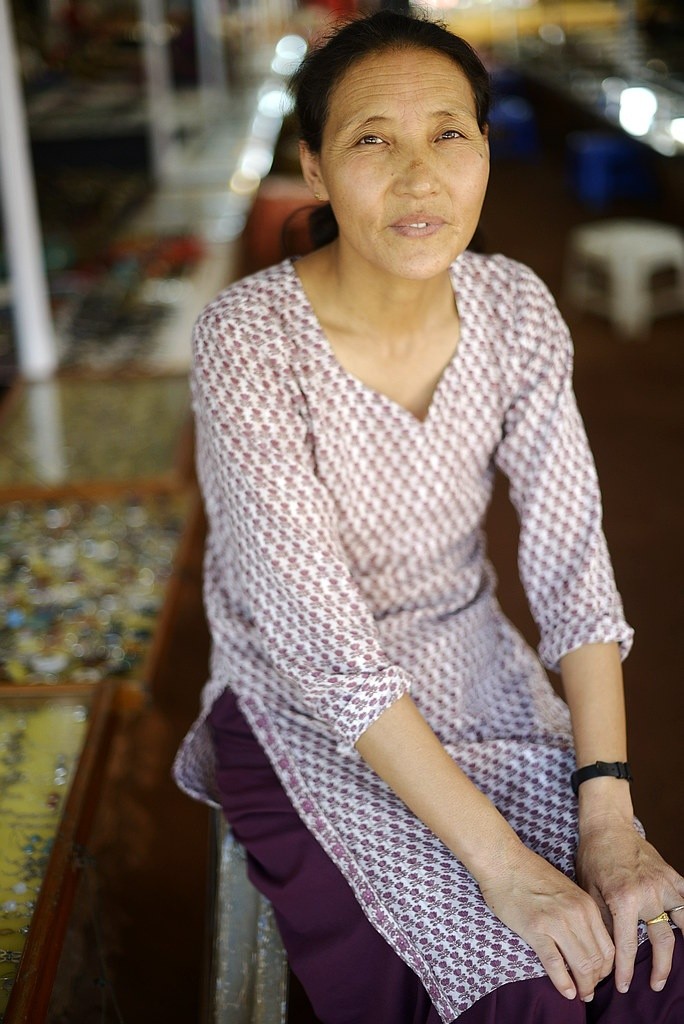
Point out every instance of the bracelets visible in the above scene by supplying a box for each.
[570,761,632,795]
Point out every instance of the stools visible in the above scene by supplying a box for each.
[567,218,684,335]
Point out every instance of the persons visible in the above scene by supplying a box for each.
[172,14,684,1024]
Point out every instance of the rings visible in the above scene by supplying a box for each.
[673,905,684,912]
[646,913,668,925]
